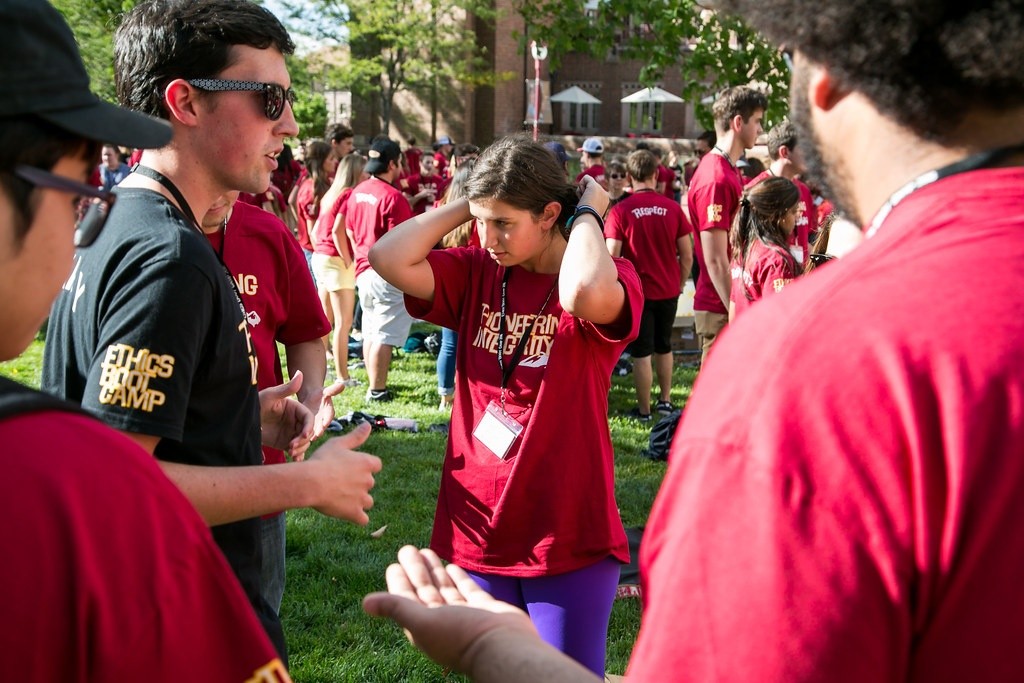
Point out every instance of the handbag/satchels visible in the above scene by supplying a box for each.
[642,407,683,461]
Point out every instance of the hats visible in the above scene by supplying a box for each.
[542,142,571,162]
[576,137,604,154]
[438,136,456,147]
[1,0,174,149]
[735,151,750,169]
[364,139,398,173]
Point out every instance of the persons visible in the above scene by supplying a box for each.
[44,0,382,683]
[687,87,767,370]
[99,144,143,193]
[799,174,862,276]
[345,136,412,404]
[204,189,331,616]
[728,177,800,327]
[367,133,645,683]
[605,151,693,420]
[310,155,370,389]
[743,122,818,271]
[0,0,292,683]
[362,1,1024,683]
[238,125,765,359]
[437,159,480,411]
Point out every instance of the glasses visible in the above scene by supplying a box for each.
[694,149,705,156]
[606,173,626,179]
[0,158,117,249]
[159,78,296,121]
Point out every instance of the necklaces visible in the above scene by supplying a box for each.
[769,168,775,176]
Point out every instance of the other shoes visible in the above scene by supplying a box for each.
[630,408,653,422]
[439,398,453,413]
[656,399,675,416]
[335,377,362,388]
[365,387,394,405]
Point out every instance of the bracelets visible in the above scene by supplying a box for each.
[566,208,604,234]
[575,205,604,224]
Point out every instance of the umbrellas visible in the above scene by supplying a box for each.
[551,86,602,128]
[622,86,686,130]
